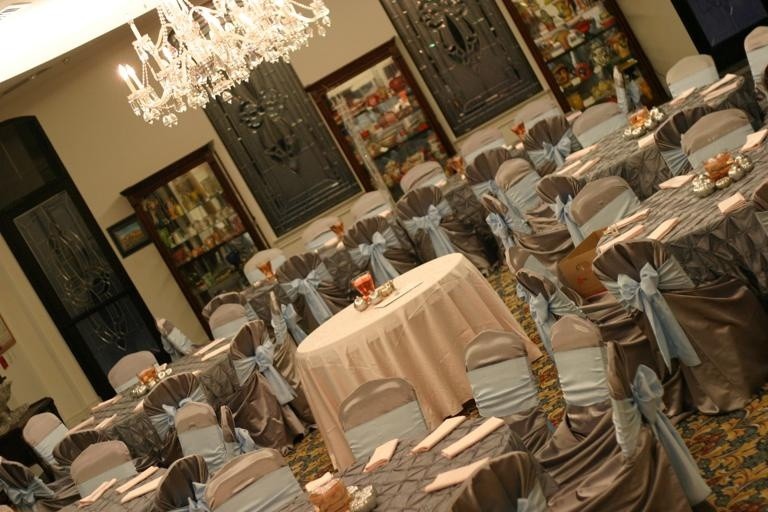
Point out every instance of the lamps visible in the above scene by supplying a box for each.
[116,0,330,128]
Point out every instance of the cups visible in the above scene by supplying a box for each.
[330,221,344,236]
[351,270,374,297]
[137,368,157,388]
[511,121,526,139]
[258,260,273,277]
